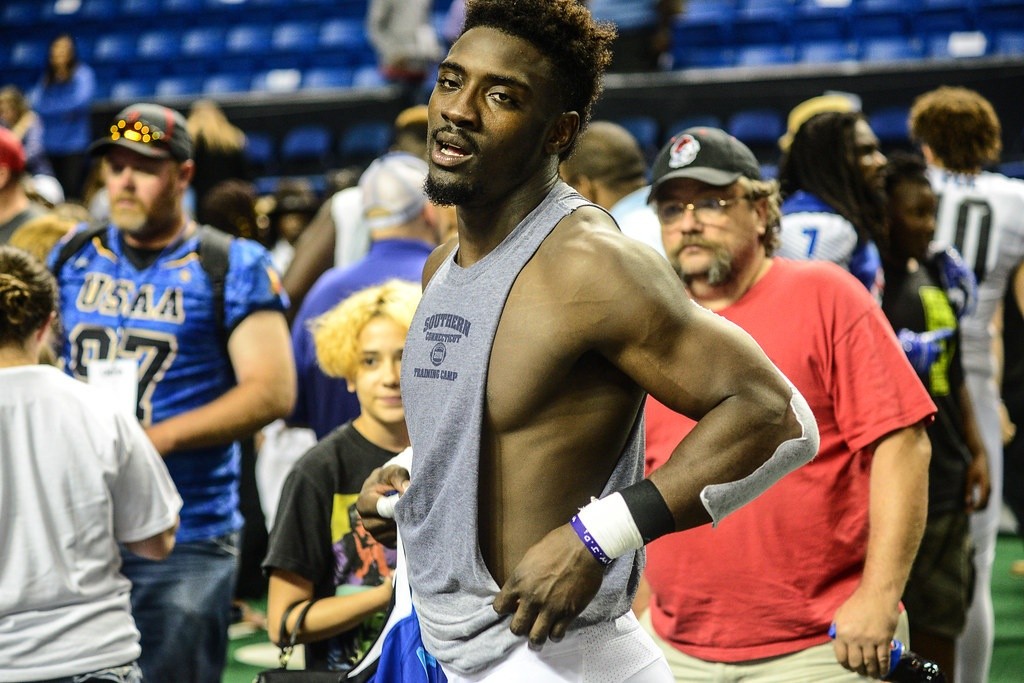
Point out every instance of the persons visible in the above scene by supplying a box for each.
[630,124,938,683]
[770,86,1024,683]
[559,120,671,266]
[1,0,471,671]
[259,278,423,683]
[40,101,299,683]
[393,0,821,683]
[0,246,185,683]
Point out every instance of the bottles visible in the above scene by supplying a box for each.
[826,624,946,683]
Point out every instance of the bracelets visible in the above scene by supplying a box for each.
[382,446,412,480]
[567,514,615,568]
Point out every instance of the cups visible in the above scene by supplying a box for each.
[85,358,138,423]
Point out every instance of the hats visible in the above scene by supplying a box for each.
[645,126,762,208]
[358,149,431,226]
[0,127,26,173]
[87,101,193,166]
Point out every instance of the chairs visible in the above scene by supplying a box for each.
[0,1,1024,214]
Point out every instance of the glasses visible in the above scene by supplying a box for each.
[657,195,753,223]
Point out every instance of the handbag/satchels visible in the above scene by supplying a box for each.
[254,598,348,683]
